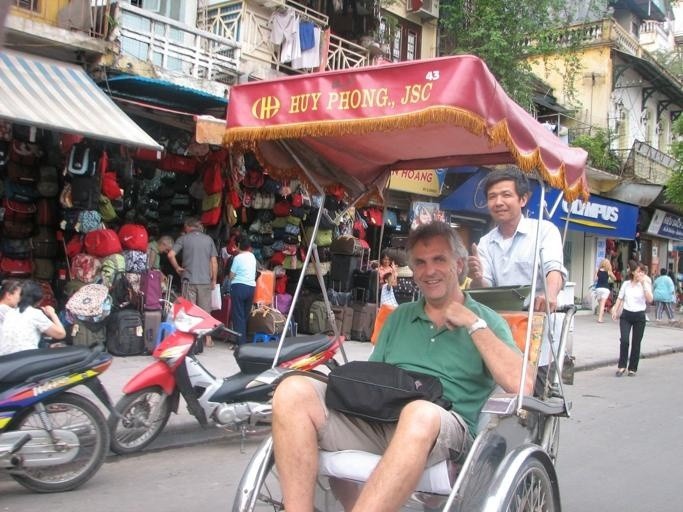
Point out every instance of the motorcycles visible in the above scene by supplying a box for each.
[106,290,345,455]
[0,343,129,495]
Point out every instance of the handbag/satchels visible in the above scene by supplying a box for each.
[325,361,452,422]
[608,277,614,283]
[0,119,444,356]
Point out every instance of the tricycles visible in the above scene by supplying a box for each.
[217,50,596,510]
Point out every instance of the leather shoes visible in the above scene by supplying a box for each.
[616,369,633,377]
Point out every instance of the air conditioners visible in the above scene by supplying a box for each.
[405,0,439,22]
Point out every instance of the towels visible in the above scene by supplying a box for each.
[299,21,315,52]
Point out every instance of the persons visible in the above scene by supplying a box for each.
[272,220,535,512]
[167,217,218,347]
[615,259,675,322]
[595,259,616,323]
[0,280,66,356]
[464,168,569,498]
[612,262,654,377]
[0,279,21,327]
[380,270,399,309]
[228,235,256,350]
[149,235,174,269]
[376,252,397,290]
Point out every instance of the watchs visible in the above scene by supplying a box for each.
[467,319,487,336]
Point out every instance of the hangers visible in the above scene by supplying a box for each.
[273,5,328,31]
